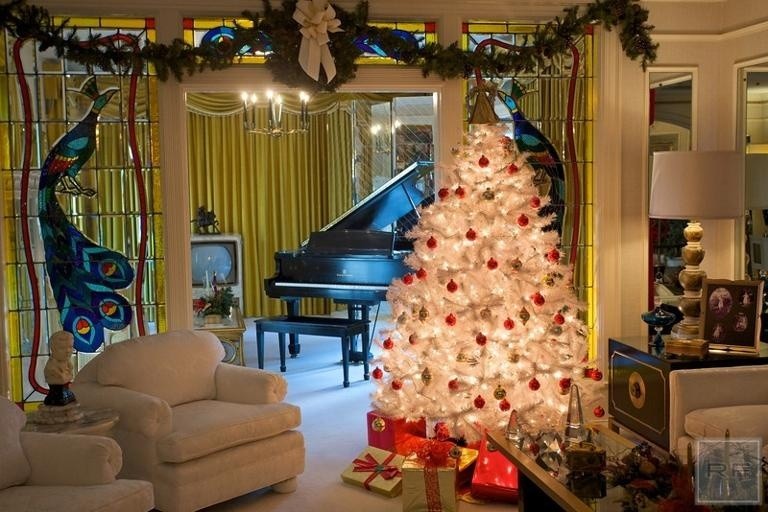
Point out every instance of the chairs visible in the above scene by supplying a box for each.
[72,329,306,512]
[669,364,768,482]
[0,396,155,512]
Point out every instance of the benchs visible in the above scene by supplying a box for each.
[253,316,371,389]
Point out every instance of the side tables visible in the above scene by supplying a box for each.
[22,408,120,434]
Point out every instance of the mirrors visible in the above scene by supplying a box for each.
[734,55,767,348]
[645,66,698,333]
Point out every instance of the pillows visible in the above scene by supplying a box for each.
[96,331,226,407]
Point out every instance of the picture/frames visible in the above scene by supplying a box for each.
[700,278,764,353]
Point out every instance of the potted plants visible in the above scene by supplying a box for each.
[202,287,238,324]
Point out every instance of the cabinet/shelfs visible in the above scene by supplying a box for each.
[608,336,768,455]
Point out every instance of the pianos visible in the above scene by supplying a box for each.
[265,160,433,362]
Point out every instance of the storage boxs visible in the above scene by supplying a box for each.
[471,435,519,502]
[367,409,426,455]
[401,452,458,512]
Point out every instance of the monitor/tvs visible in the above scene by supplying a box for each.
[189,244,235,285]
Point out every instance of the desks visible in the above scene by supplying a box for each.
[193,297,246,366]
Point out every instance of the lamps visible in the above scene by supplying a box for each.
[649,149,740,339]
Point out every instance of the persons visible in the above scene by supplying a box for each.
[44,331,74,385]
